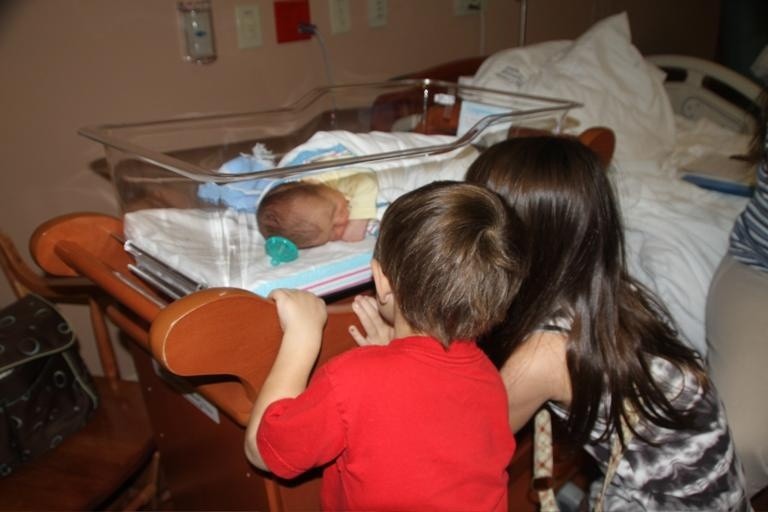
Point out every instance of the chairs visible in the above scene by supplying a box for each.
[0,233,161,512]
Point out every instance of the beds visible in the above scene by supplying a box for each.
[29,75,615,512]
[481,54,767,376]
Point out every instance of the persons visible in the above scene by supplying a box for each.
[243,178,533,509]
[703,82,768,501]
[462,133,757,511]
[255,163,383,253]
